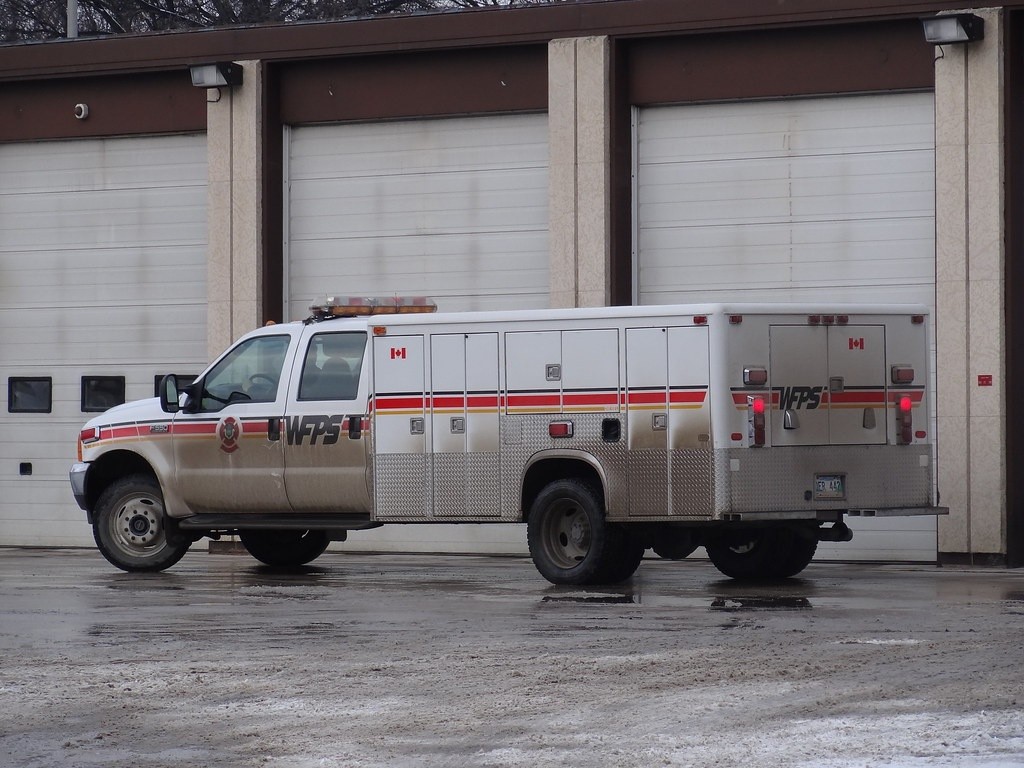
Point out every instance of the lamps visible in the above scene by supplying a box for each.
[921,13,986,47]
[187,61,244,89]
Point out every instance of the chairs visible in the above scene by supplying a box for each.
[306,357,357,400]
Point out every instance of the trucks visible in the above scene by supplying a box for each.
[72,295,948,587]
